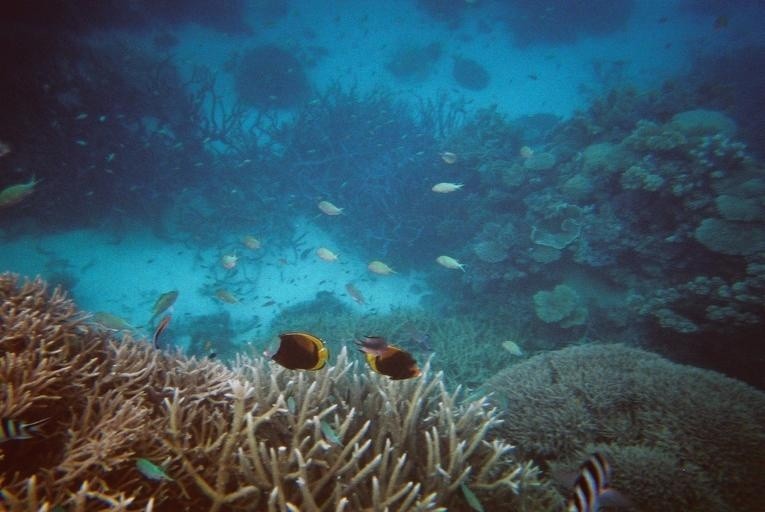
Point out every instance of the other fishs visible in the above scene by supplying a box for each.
[0,0,536,382]
